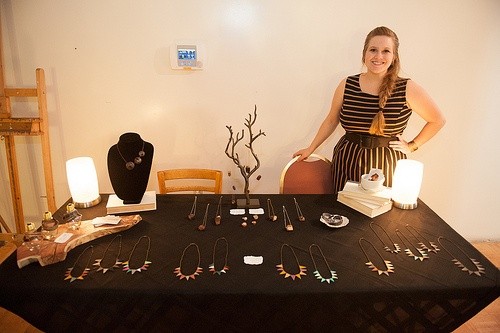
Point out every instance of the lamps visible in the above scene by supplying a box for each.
[391,159,424,210]
[66,157,101,209]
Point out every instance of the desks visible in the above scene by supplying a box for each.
[0,194,500,333]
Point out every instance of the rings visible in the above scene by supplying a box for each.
[397,144,399,148]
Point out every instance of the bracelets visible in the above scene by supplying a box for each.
[408,140,419,153]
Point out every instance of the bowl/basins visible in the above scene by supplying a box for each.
[361,174,385,192]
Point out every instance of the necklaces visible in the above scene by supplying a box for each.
[438,236,486,276]
[369,222,401,253]
[64,246,93,282]
[188,196,223,231]
[309,244,338,283]
[116,141,145,170]
[282,206,293,231]
[267,199,277,221]
[359,237,395,277]
[209,237,230,275]
[406,223,441,253]
[294,198,305,221]
[93,235,124,274]
[396,228,430,262]
[276,244,307,281]
[174,243,203,281]
[122,236,152,275]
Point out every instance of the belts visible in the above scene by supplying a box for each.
[345,132,398,149]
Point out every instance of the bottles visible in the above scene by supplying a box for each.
[66,203,82,229]
[25,222,41,250]
[40,211,59,240]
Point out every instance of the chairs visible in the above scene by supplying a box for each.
[279,154,334,194]
[158,168,223,194]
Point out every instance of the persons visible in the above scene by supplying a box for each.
[292,26,447,195]
